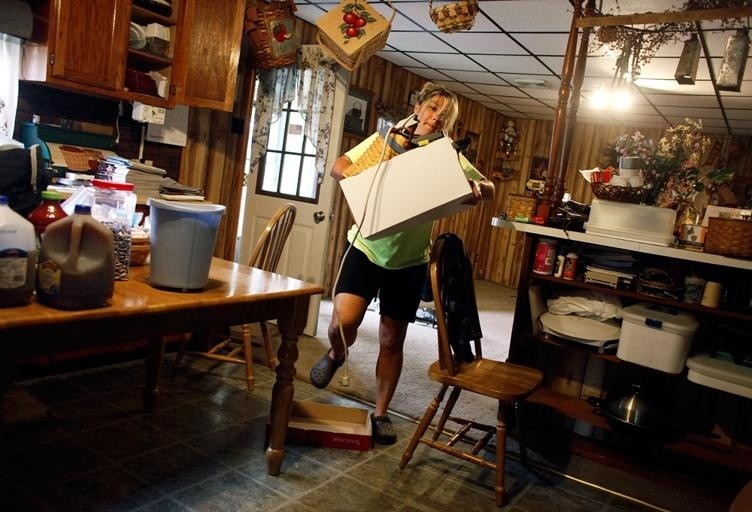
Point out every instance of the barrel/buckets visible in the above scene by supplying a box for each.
[35,205,114,310]
[148,196,226,291]
[0,195,36,309]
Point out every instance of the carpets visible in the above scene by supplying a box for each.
[224,292,514,445]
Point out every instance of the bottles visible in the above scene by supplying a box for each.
[29,190,68,233]
[532,238,579,281]
[91,180,136,282]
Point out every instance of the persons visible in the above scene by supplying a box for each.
[308,82,496,444]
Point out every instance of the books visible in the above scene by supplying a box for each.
[584,264,637,289]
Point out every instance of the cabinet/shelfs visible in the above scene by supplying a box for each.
[497,231,752,493]
[19,0,246,113]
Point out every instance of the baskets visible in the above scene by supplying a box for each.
[702,217,752,259]
[247,4,298,68]
[590,181,656,206]
[129,242,150,265]
[430,0,480,32]
[505,194,541,220]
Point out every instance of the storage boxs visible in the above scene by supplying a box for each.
[615,302,699,374]
[703,204,752,260]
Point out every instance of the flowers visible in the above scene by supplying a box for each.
[597,116,735,207]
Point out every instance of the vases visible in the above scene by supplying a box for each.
[668,197,700,236]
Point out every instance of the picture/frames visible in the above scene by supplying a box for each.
[342,89,372,141]
[407,91,417,107]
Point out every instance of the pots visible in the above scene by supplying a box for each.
[588,384,646,428]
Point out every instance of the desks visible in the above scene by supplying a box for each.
[0,259,324,475]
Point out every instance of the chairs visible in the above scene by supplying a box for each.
[172,205,298,391]
[397,231,544,507]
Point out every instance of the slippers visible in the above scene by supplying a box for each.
[371,413,397,444]
[310,347,349,390]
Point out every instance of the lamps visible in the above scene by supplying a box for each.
[714,27,751,91]
[587,30,640,112]
[674,31,701,85]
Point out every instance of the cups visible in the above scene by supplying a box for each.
[683,275,723,309]
